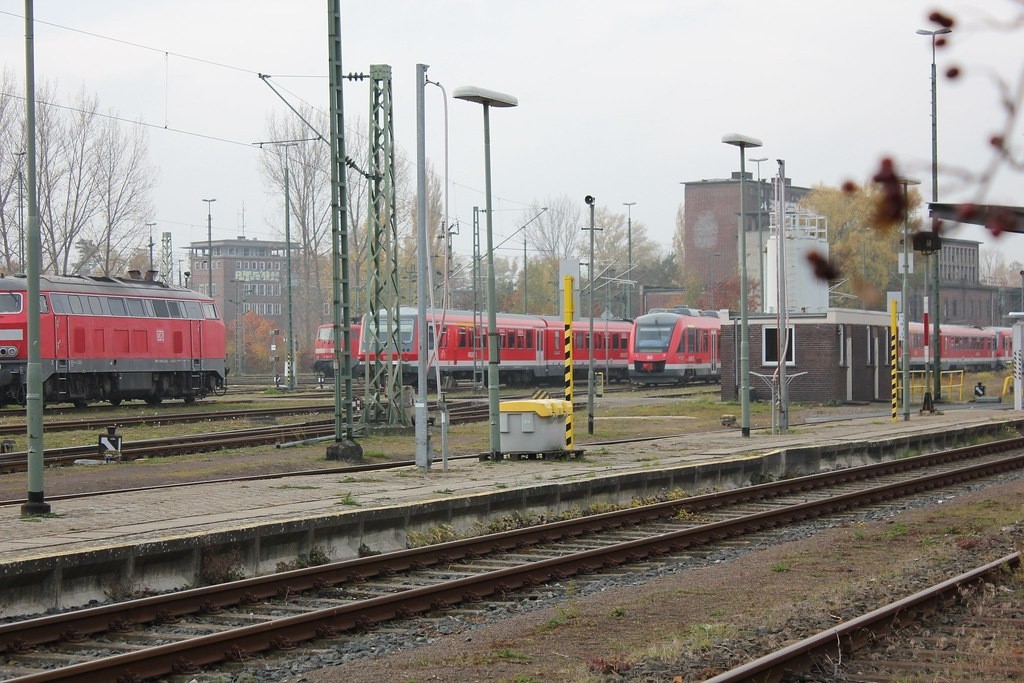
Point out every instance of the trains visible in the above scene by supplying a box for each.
[0,268,231,408]
[312,316,362,378]
[356,303,635,394]
[626,304,1012,390]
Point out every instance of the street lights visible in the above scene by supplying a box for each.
[748,158,770,313]
[877,174,920,421]
[623,202,637,320]
[452,85,519,463]
[202,199,216,298]
[275,141,298,391]
[721,131,764,438]
[145,223,157,280]
[915,28,952,399]
[179,260,185,286]
[710,253,722,310]
